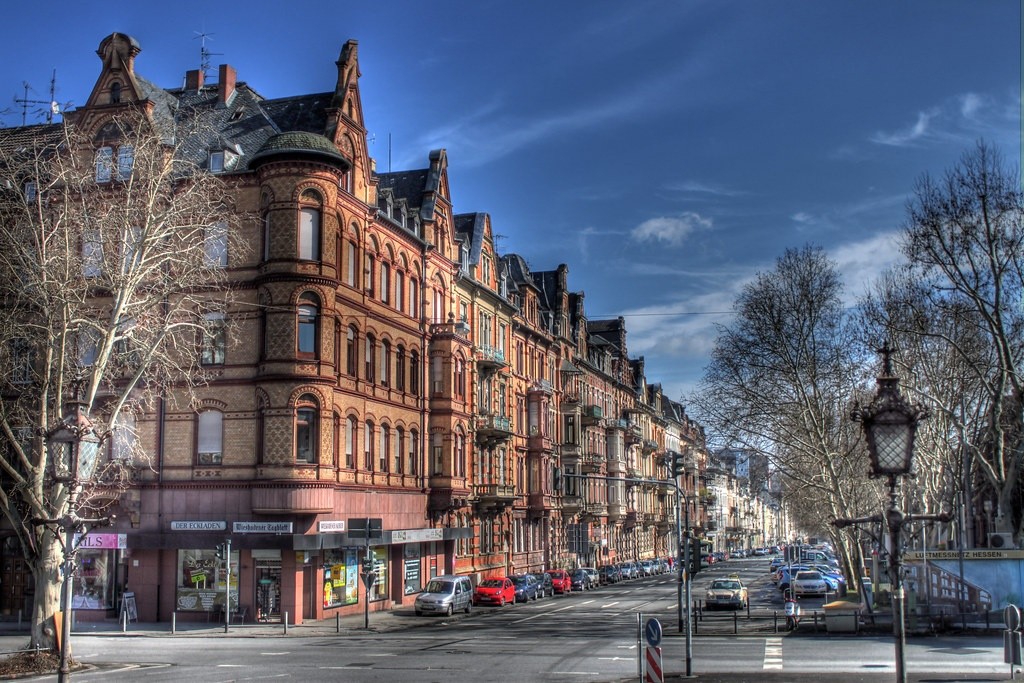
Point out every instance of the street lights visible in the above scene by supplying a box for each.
[29,376,123,683]
[846,335,955,683]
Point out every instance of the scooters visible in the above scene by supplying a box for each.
[784,596,802,631]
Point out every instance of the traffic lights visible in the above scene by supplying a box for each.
[362,556,370,573]
[215,543,226,560]
[371,551,378,571]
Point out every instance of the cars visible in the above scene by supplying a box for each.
[580,567,600,588]
[472,577,516,606]
[504,573,539,602]
[567,569,592,592]
[547,570,572,593]
[635,541,847,593]
[532,572,555,598]
[613,563,623,581]
[598,564,619,584]
[620,561,638,580]
[790,570,828,597]
[704,574,748,610]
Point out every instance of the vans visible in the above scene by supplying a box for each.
[415,575,473,616]
